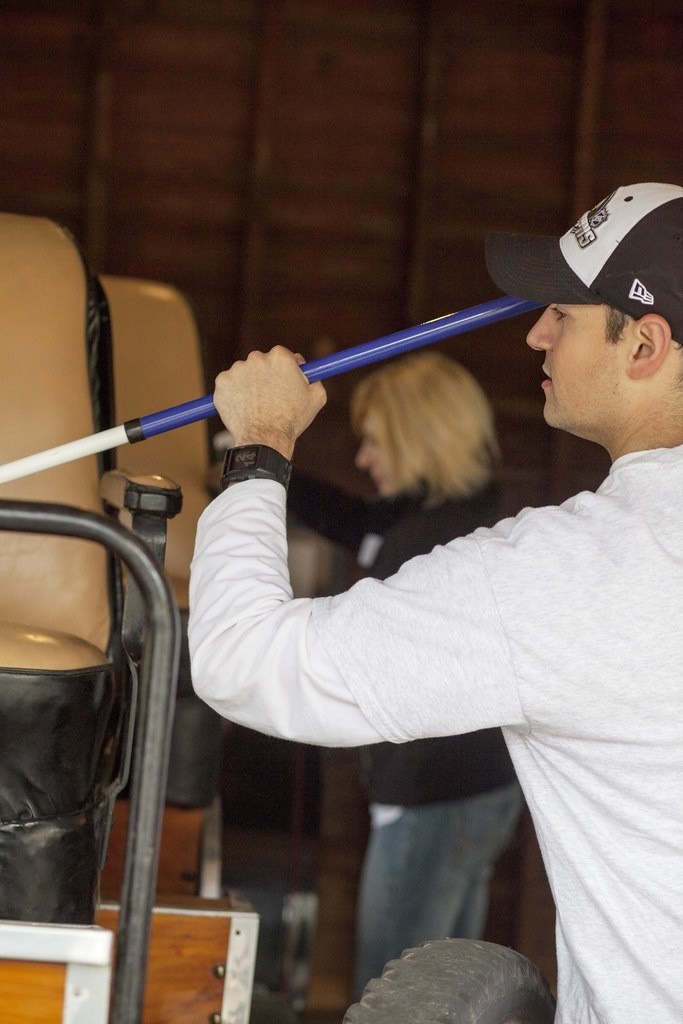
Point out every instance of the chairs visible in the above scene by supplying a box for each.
[0,214,219,923]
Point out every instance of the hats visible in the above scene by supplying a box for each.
[484,181,683,346]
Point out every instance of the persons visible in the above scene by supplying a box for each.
[187,177,683,1024]
[319,348,528,1010]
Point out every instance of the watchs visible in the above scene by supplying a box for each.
[219,443,294,493]
